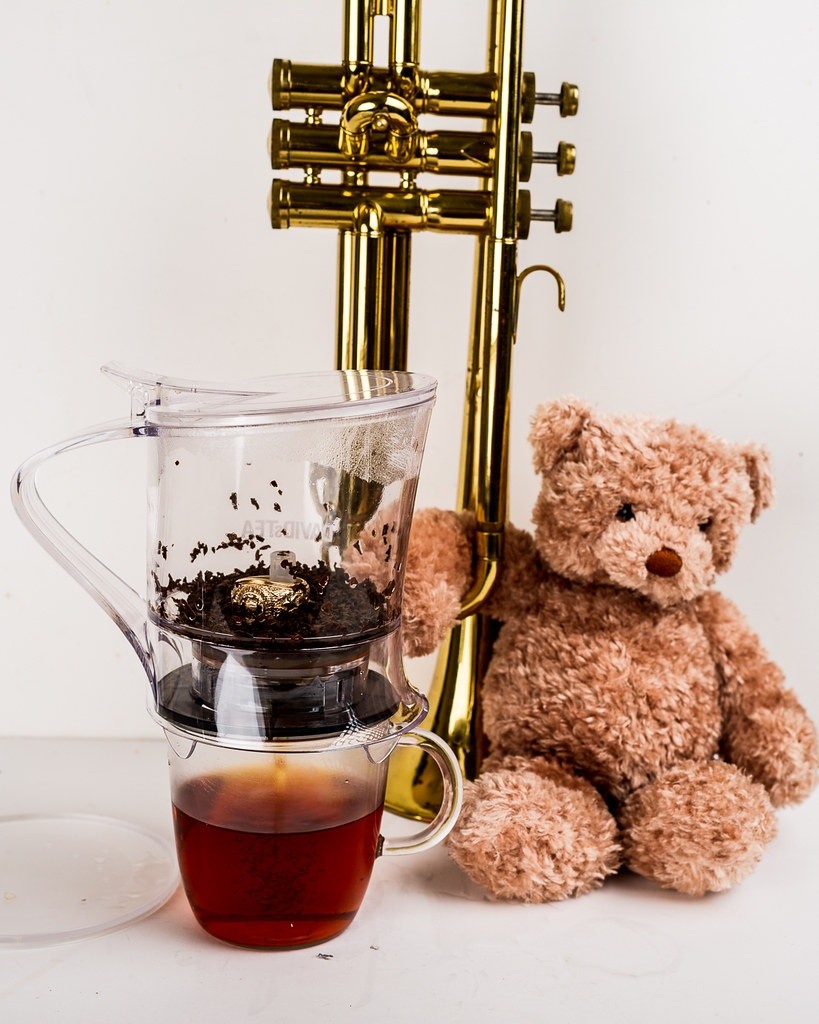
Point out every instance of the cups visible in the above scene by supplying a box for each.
[147,689,463,949]
[10,369,439,732]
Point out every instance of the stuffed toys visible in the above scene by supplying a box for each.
[340,397,819,903]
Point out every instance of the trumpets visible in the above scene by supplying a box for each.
[270,0,580,826]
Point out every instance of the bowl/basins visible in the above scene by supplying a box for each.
[0,737,181,946]
